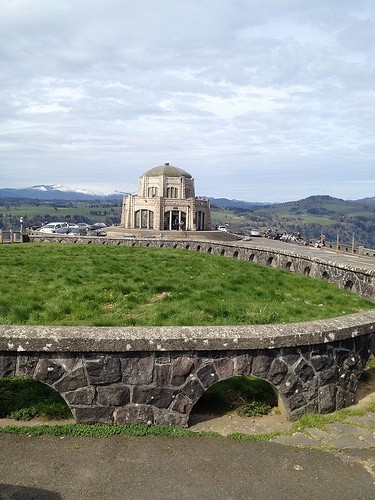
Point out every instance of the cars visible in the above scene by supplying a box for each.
[35,221,106,237]
[215,225,283,240]
